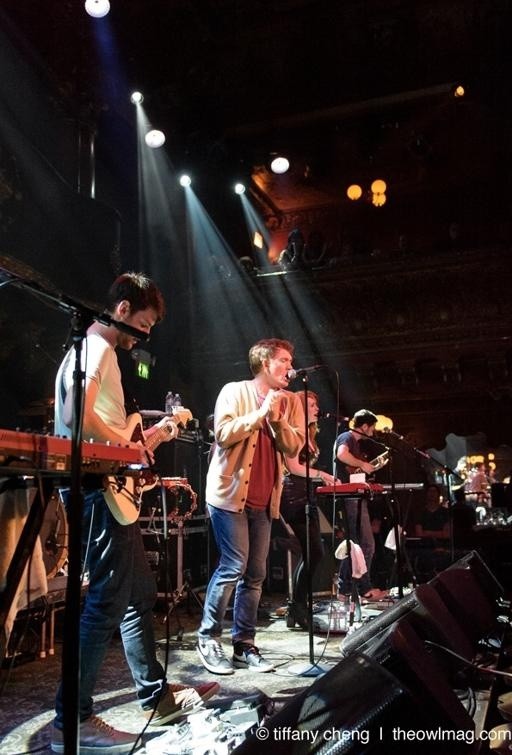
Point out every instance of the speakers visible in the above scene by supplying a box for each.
[366,618,475,749]
[341,583,479,689]
[426,559,498,642]
[231,650,469,755]
[448,538,506,614]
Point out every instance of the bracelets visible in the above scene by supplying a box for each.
[314,468,321,477]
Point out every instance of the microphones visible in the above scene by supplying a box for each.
[384,427,403,440]
[322,411,350,422]
[287,364,321,379]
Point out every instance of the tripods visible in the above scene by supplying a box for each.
[161,539,204,625]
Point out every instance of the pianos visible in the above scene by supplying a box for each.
[1,426,140,478]
[316,483,425,496]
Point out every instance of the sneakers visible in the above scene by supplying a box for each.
[143,681,220,726]
[51,714,141,755]
[337,593,348,601]
[360,588,390,605]
[197,637,275,674]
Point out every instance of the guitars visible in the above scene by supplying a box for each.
[346,448,391,482]
[97,407,194,526]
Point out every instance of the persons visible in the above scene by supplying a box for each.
[278,390,343,634]
[49,271,223,752]
[414,484,454,583]
[332,407,391,604]
[194,337,305,676]
[447,455,500,549]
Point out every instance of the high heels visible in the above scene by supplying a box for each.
[286,601,309,630]
